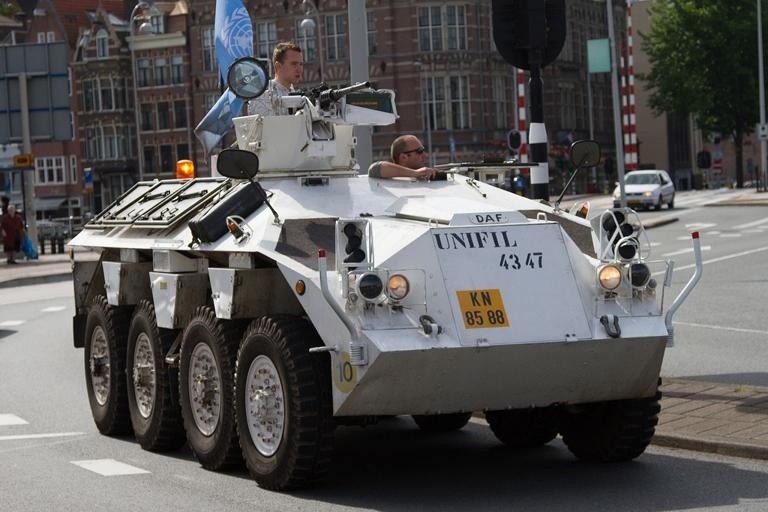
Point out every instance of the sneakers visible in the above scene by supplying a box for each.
[7,260,17,264]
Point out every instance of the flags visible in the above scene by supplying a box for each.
[193,0,254,164]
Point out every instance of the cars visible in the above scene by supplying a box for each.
[613,168,676,211]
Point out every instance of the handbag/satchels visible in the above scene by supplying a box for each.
[23,238,36,257]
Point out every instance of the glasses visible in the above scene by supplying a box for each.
[403,145,426,155]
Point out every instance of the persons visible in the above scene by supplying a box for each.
[367,135,438,179]
[248,42,304,114]
[1,205,25,264]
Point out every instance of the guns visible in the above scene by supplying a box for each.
[288,81,371,115]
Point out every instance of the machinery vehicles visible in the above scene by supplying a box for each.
[65,58,702,494]
